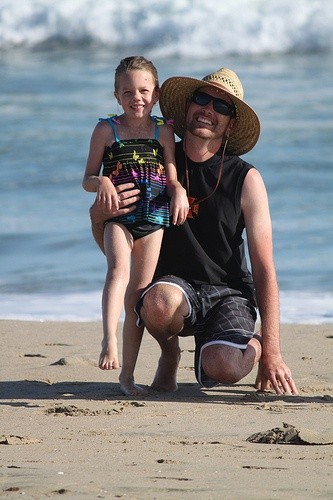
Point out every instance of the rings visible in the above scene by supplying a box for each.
[286,376,292,382]
[118,194,121,200]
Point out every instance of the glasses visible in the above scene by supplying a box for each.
[191,90,237,120]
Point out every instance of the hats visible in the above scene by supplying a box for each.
[159,67,261,156]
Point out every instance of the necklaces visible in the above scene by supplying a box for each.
[194,142,216,161]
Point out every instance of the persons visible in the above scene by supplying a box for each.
[89,67,299,395]
[82,56,190,397]
